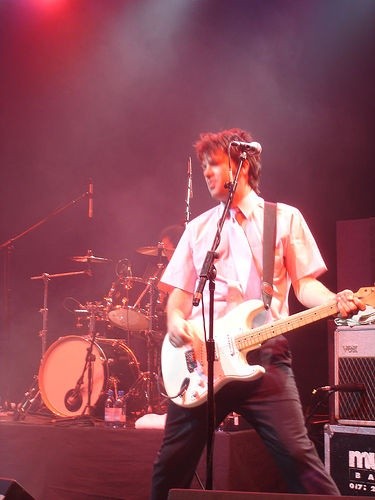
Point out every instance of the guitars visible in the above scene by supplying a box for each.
[160,286,375,409]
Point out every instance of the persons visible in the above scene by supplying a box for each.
[151,127,367,500]
[160,222,186,266]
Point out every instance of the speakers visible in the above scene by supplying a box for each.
[167,488,375,500]
[333,325,375,427]
[0,478,35,500]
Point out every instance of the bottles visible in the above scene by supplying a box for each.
[104,390,114,428]
[113,390,127,428]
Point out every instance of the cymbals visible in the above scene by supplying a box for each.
[71,255,112,263]
[136,246,167,256]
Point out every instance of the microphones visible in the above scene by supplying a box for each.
[126,262,132,289]
[187,158,193,198]
[67,376,83,407]
[319,384,364,392]
[89,178,94,217]
[77,302,90,320]
[231,141,262,156]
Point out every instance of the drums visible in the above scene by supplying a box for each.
[107,276,159,333]
[38,335,141,417]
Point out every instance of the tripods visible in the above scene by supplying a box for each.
[114,247,166,409]
